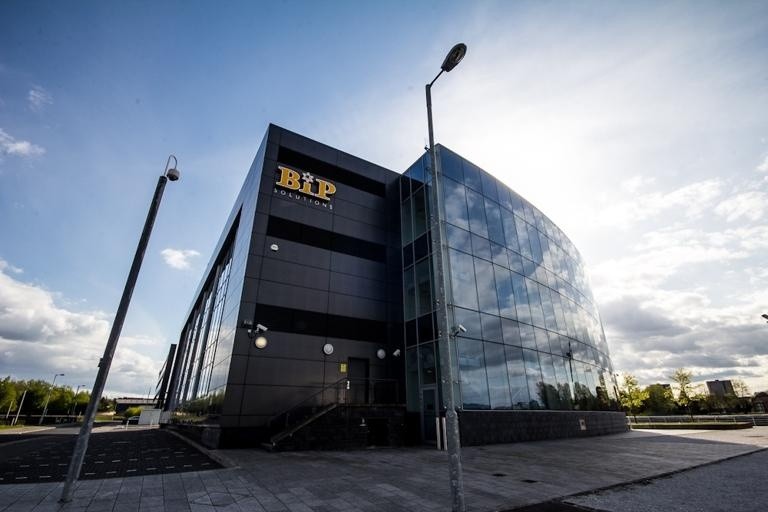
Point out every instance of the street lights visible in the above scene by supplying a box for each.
[74,384,86,413]
[761,313,768,322]
[58,152,181,512]
[36,372,65,425]
[423,41,474,511]
